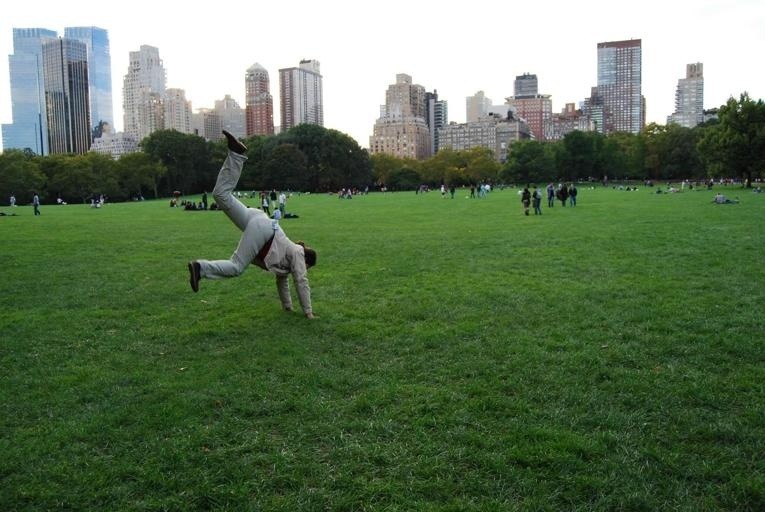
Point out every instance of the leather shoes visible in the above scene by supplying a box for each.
[188,261,201,293]
[221,129,247,156]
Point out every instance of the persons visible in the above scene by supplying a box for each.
[32,191,41,216]
[8,196,16,215]
[188,130,316,320]
